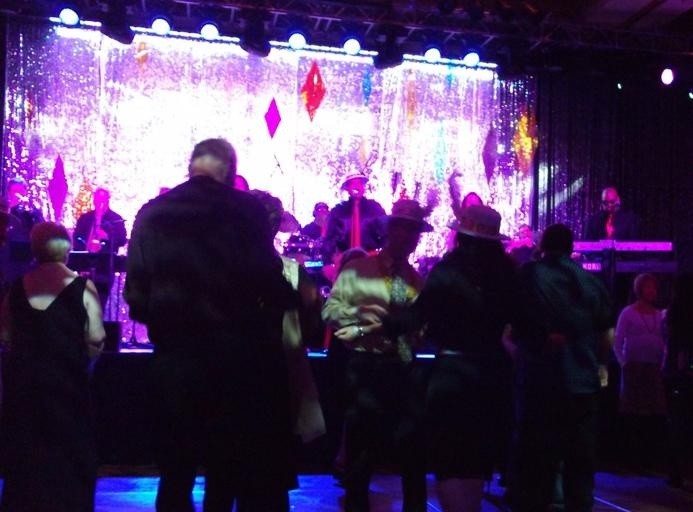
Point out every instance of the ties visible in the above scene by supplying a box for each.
[604,215,615,240]
[389,267,417,365]
[349,197,365,252]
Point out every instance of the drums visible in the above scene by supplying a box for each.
[283,234,325,261]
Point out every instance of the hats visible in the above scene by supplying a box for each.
[376,197,434,237]
[446,205,513,244]
[338,164,369,189]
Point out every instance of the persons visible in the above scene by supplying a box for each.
[0,135,692,511]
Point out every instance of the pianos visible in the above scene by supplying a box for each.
[66,250,127,272]
[572,240,679,273]
